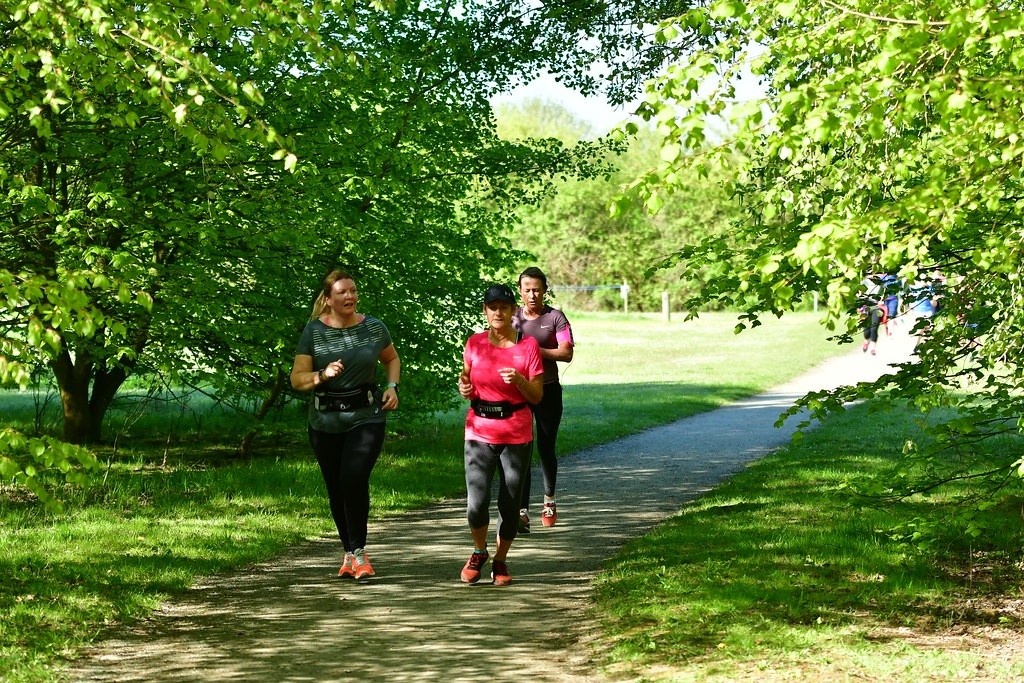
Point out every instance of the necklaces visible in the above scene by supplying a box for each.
[492,329,510,342]
[329,313,355,329]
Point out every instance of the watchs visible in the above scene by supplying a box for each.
[385,382,401,394]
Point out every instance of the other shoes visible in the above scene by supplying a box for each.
[863,340,870,352]
[871,349,876,356]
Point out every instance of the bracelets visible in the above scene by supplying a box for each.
[318,368,326,383]
[516,372,526,394]
[456,381,464,386]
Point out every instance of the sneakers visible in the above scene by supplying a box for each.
[460,550,489,583]
[490,559,512,586]
[542,501,558,528]
[338,551,357,578]
[518,509,531,534]
[351,548,375,580]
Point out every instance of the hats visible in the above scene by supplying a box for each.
[483,284,516,304]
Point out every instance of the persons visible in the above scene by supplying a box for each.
[961,274,981,351]
[458,283,544,586]
[858,270,888,355]
[510,266,575,533]
[909,264,947,350]
[884,273,898,325]
[290,269,400,579]
